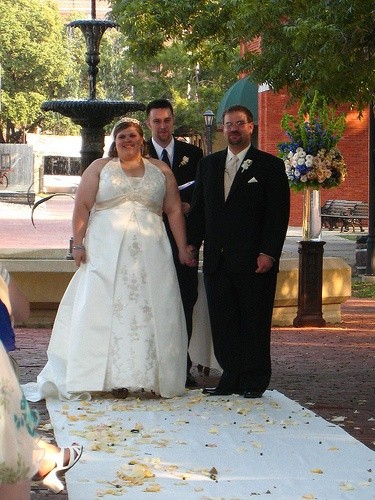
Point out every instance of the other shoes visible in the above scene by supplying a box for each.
[186,374,198,386]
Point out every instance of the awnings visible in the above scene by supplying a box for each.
[216,76,259,129]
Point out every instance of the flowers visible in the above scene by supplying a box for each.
[277,90,348,194]
[178,155,189,168]
[240,159,253,174]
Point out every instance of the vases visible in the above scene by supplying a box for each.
[300,188,322,242]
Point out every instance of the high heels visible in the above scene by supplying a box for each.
[41,443,83,494]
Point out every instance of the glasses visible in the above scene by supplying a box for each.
[224,121,251,130]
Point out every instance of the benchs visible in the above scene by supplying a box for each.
[322,199,369,233]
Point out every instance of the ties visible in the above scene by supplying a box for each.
[162,149,171,169]
[225,156,239,200]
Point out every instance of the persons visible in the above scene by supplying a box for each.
[145,99,205,389]
[0,266,84,500]
[21,118,195,403]
[185,105,290,400]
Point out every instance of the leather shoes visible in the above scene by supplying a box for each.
[244,391,263,398]
[203,387,227,395]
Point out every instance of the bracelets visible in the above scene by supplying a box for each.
[72,245,86,250]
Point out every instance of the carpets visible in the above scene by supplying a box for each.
[45,388,375,500]
